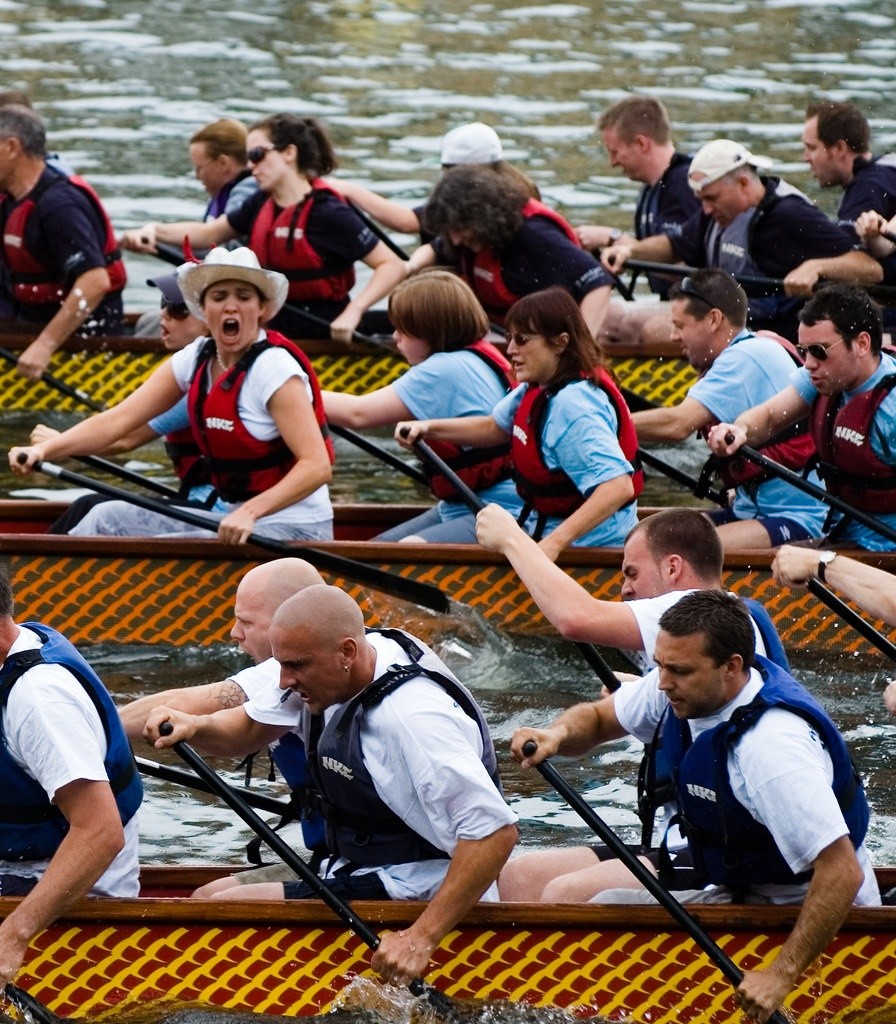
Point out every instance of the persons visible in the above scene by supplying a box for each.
[124,114,404,348]
[709,285,896,554]
[142,583,519,989]
[770,544,896,716]
[318,270,539,547]
[7,245,333,544]
[800,97,896,343]
[476,503,792,904]
[602,142,884,342]
[28,260,211,532]
[320,122,613,341]
[575,97,714,302]
[0,87,126,338]
[0,560,145,1001]
[630,269,829,561]
[394,287,640,547]
[508,589,883,1024]
[117,558,329,899]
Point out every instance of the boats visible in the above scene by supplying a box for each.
[1,498,896,657]
[0,300,896,435]
[0,862,895,1023]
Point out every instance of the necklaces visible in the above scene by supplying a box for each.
[215,348,229,371]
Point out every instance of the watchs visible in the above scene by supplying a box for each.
[818,550,837,583]
[608,226,622,247]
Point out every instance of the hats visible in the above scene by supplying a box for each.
[144,259,204,303]
[441,122,529,165]
[687,139,774,190]
[182,245,289,324]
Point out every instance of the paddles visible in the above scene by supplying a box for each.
[4,982,66,1024]
[725,434,896,545]
[591,246,636,302]
[343,195,409,261]
[133,754,298,820]
[67,450,183,500]
[572,640,621,694]
[399,427,646,677]
[875,219,896,247]
[523,740,792,1024]
[803,576,896,665]
[607,254,896,314]
[635,443,723,506]
[159,722,461,1019]
[325,421,432,487]
[17,451,452,617]
[0,346,107,414]
[142,235,409,363]
[489,320,667,417]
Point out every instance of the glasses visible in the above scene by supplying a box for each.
[794,334,854,360]
[244,145,276,163]
[161,296,190,320]
[505,332,547,345]
